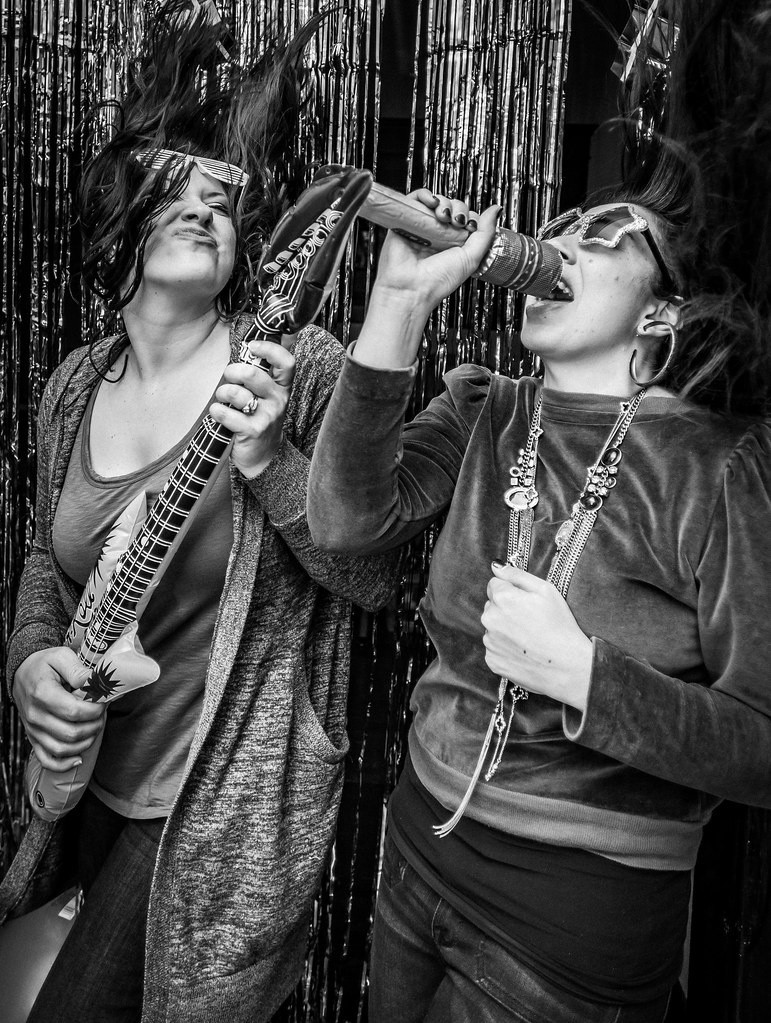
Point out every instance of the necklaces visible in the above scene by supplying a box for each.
[428,377,653,839]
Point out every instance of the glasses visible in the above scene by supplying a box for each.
[128,148,246,226]
[535,206,677,299]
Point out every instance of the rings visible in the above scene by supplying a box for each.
[242,393,259,417]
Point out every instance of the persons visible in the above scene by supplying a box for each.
[304,192,771,1022]
[0,0,405,1023]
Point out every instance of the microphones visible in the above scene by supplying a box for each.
[351,176,562,297]
